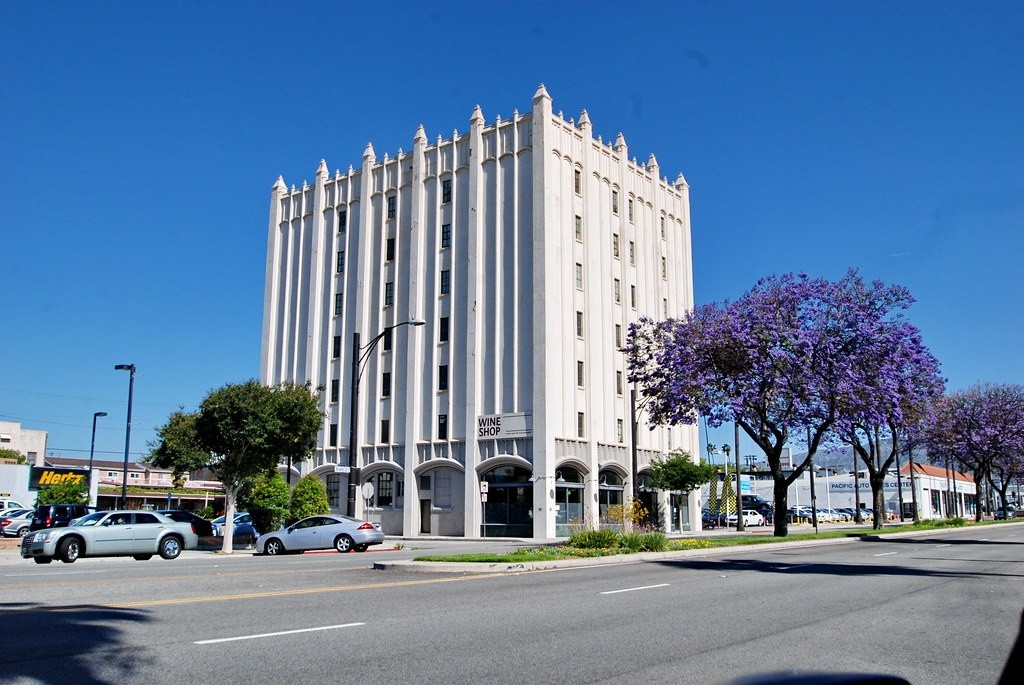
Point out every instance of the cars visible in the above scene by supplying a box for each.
[210,511,260,538]
[0,509,36,537]
[766,505,896,526]
[726,510,764,527]
[154,510,212,536]
[702,508,726,526]
[68,513,115,527]
[255,514,385,555]
[20,509,199,564]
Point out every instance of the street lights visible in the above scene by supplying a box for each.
[115,364,136,509]
[719,451,729,528]
[347,318,427,518]
[819,467,831,523]
[87,412,107,500]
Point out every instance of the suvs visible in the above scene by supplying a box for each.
[30,505,89,531]
[994,507,1015,521]
[736,495,773,512]
[0,493,25,509]
